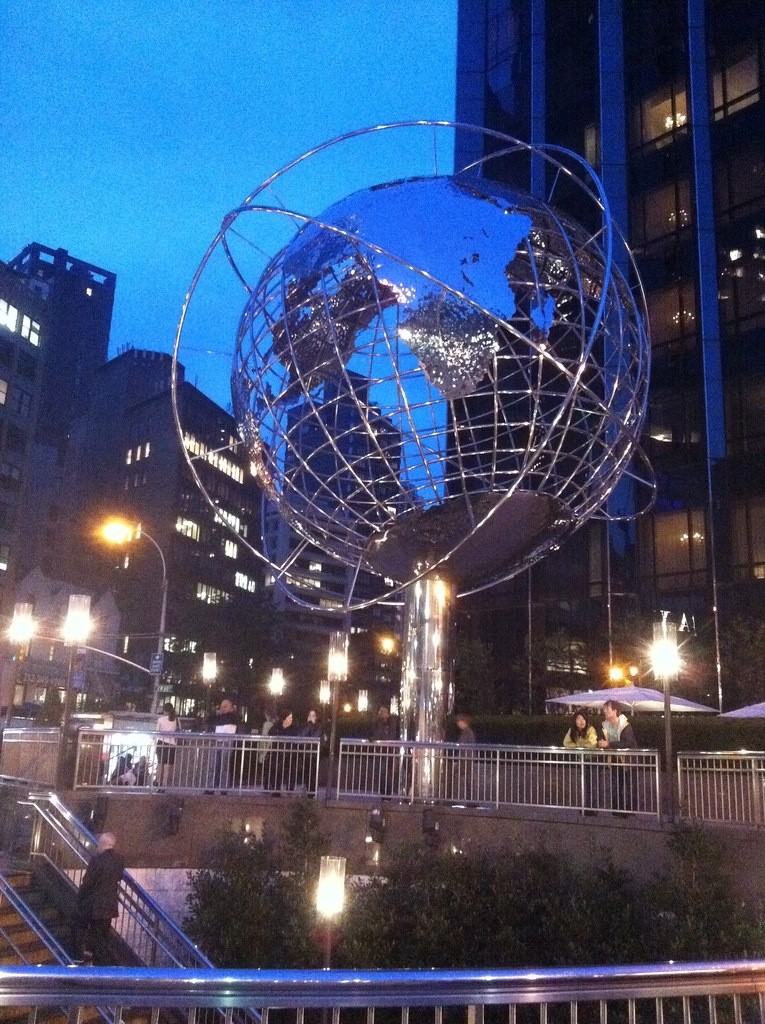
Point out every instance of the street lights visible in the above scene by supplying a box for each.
[103,522,167,712]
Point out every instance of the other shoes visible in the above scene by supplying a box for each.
[271,783,281,797]
[203,790,215,796]
[466,801,480,808]
[449,801,465,806]
[218,791,228,796]
[161,788,165,793]
[403,799,416,804]
[579,806,599,817]
[612,810,636,819]
[308,785,318,798]
[263,785,270,794]
[72,954,85,963]
[382,798,392,803]
[155,788,161,794]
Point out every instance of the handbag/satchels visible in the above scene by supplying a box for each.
[173,729,186,746]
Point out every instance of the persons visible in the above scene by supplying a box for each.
[110,753,149,788]
[201,698,240,796]
[258,708,296,797]
[155,703,179,794]
[596,700,642,817]
[443,712,481,809]
[72,831,123,963]
[560,711,605,819]
[296,710,322,798]
[366,704,401,802]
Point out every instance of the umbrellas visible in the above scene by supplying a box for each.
[546,686,721,717]
[717,702,765,718]
